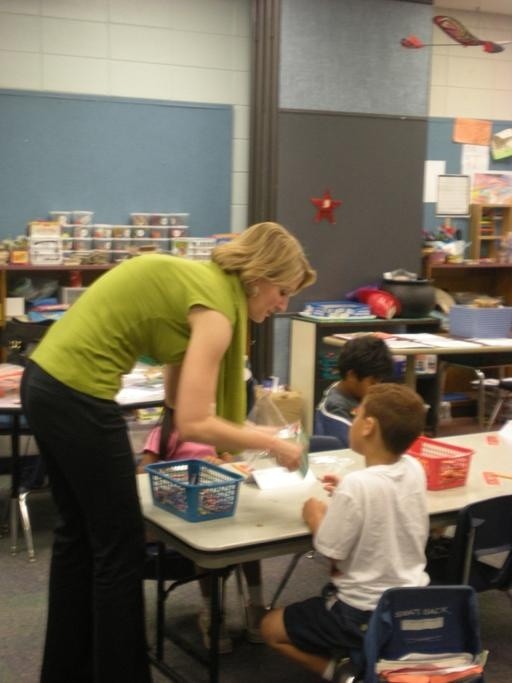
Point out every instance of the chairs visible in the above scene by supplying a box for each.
[10,475,52,564]
[340,494,511,682]
[307,402,353,451]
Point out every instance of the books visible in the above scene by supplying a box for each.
[375,651,484,683]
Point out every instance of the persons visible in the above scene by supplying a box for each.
[19,222,318,682]
[260,383,430,683]
[314,336,394,449]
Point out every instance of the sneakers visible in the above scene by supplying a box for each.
[242,608,266,644]
[334,658,354,683]
[199,614,232,654]
[427,537,454,563]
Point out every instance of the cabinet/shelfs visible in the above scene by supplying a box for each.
[469,202,512,261]
[0,263,122,363]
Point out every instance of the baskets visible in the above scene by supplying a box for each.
[405,437,474,492]
[144,460,244,521]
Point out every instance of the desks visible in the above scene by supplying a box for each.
[319,329,512,393]
[133,429,512,682]
[287,312,442,452]
[0,360,251,535]
[425,260,512,279]
